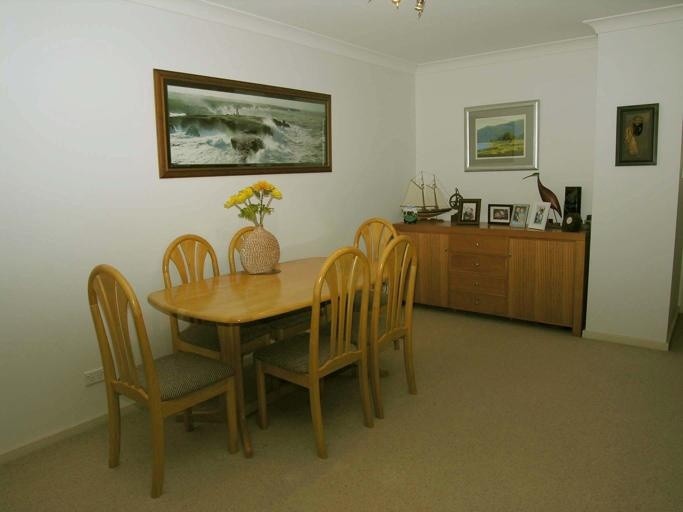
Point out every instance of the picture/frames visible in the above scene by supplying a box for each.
[457,198,551,231]
[613,102,659,166]
[461,99,540,171]
[152,67,333,179]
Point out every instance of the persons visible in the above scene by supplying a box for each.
[513,206,525,223]
[494,209,508,220]
[463,205,475,221]
[537,209,543,224]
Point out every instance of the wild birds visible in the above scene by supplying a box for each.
[522,173,562,224]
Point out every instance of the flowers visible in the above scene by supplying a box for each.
[223,180,283,224]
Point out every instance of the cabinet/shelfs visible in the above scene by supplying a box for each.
[391,223,587,337]
